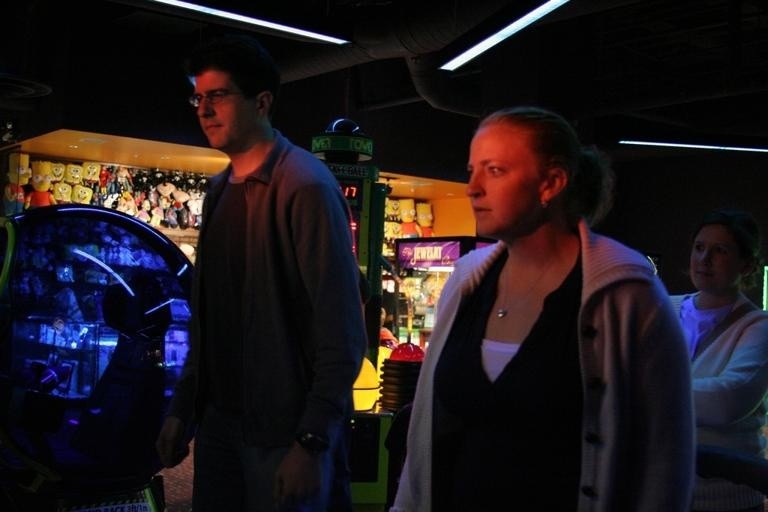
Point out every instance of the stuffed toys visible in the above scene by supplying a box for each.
[5,152,205,233]
[383,193,439,260]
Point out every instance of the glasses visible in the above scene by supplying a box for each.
[189,90,244,107]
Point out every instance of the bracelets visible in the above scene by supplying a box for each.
[295,430,331,459]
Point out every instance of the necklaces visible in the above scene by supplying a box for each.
[496,241,562,321]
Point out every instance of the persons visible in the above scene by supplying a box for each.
[662,212,766,508]
[385,103,699,512]
[157,43,366,508]
